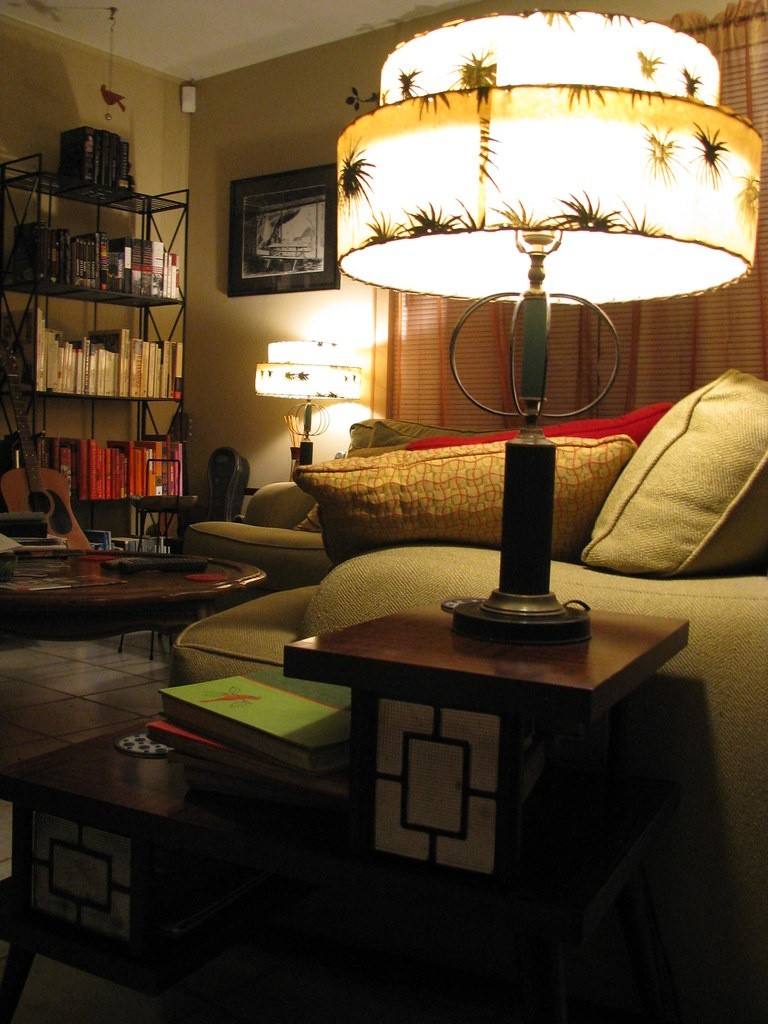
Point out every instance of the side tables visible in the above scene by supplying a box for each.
[1,595,688,1022]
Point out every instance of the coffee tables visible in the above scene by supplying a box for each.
[0,550,269,622]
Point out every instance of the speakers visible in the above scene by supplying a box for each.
[182,86,196,113]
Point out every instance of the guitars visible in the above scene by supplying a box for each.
[0,339,95,557]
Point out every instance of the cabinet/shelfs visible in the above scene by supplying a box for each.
[1,153,190,554]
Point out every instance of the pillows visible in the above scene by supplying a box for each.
[582,367,767,578]
[293,501,323,532]
[292,434,637,566]
[368,419,503,449]
[406,401,675,450]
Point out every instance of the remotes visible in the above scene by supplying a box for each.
[100,558,125,570]
[117,558,208,575]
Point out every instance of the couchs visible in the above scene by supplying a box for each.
[182,481,334,613]
[171,544,767,946]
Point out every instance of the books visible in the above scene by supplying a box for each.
[0,433,188,502]
[82,529,171,553]
[14,221,181,301]
[56,125,132,202]
[0,307,184,401]
[144,666,368,799]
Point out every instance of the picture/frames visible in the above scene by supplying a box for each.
[228,163,340,297]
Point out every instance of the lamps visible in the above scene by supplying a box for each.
[254,340,367,466]
[338,13,763,645]
